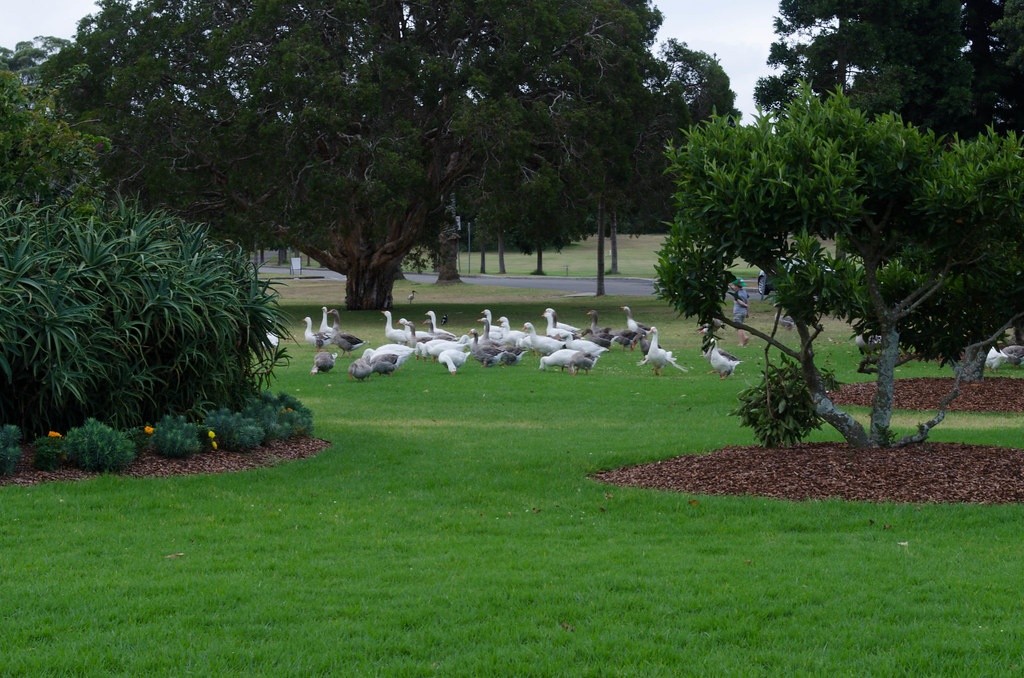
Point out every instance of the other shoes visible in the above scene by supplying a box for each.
[735,337,749,346]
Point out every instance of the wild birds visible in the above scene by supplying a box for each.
[985,346,1024,374]
[302,306,742,384]
[407,291,418,304]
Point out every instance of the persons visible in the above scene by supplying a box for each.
[728,275,750,345]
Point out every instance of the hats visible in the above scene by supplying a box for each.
[732,277,748,287]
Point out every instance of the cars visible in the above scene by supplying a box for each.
[757,257,838,296]
